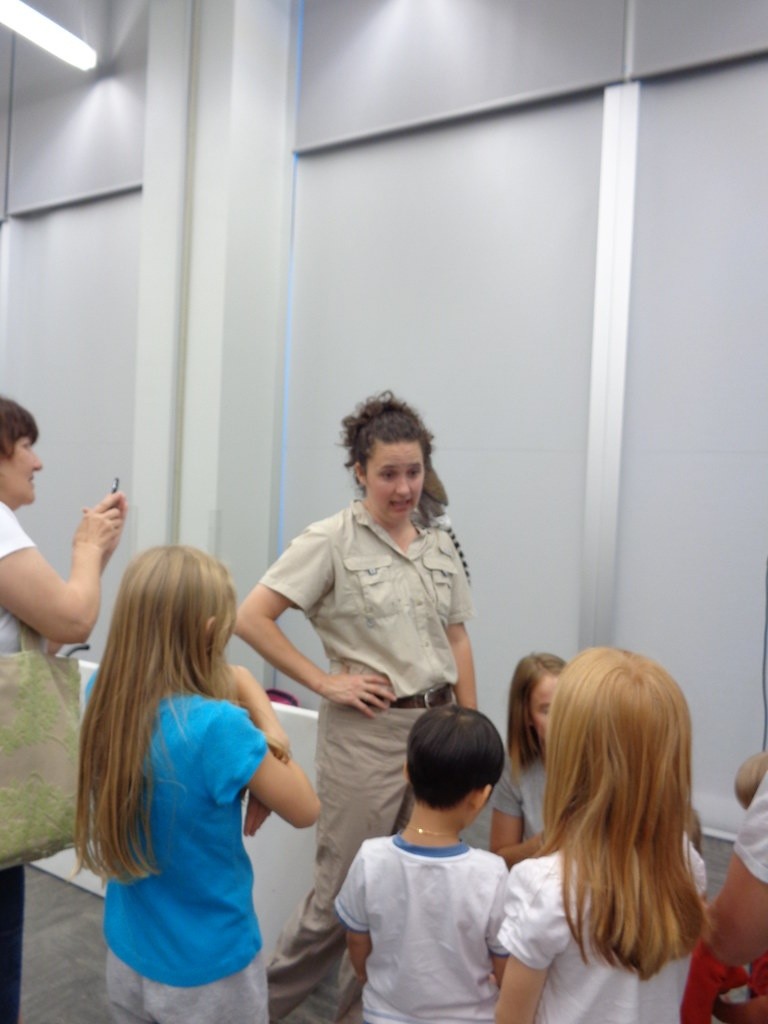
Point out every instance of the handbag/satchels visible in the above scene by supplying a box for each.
[0,619,81,871]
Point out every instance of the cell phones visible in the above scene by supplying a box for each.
[111,477,118,494]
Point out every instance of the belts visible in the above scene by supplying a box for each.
[390,684,452,709]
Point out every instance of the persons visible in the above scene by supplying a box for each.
[491,653,567,872]
[0,397,128,1023]
[497,648,724,1024]
[73,544,321,1023]
[334,707,511,1024]
[688,750,768,1023]
[231,390,476,1019]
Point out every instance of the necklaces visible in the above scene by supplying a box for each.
[406,824,459,837]
